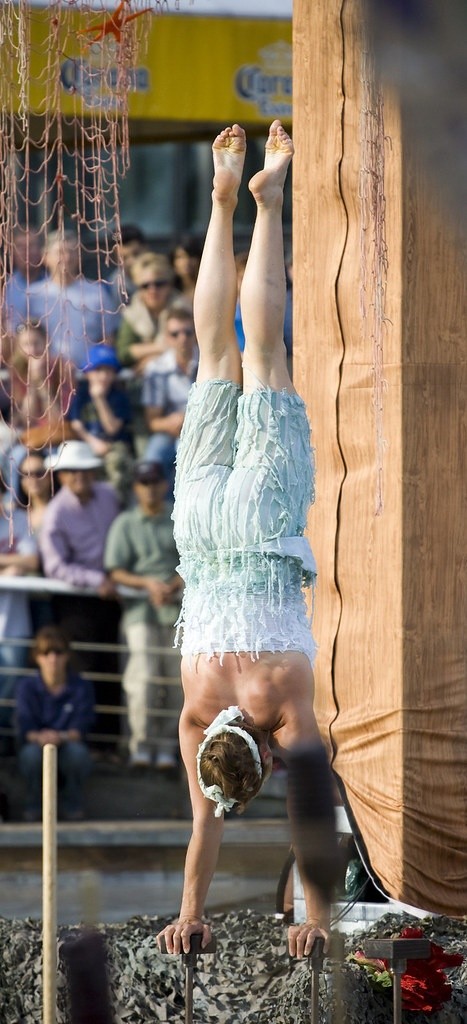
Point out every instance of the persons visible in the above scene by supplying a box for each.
[156,118,336,959]
[0,220,300,826]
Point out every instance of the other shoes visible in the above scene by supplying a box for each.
[157,754,179,772]
[130,751,150,772]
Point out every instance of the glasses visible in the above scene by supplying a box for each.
[20,469,45,483]
[167,329,194,338]
[139,281,167,294]
[36,644,70,659]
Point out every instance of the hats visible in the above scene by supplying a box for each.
[129,459,168,484]
[80,345,122,373]
[129,253,175,286]
[43,439,104,475]
